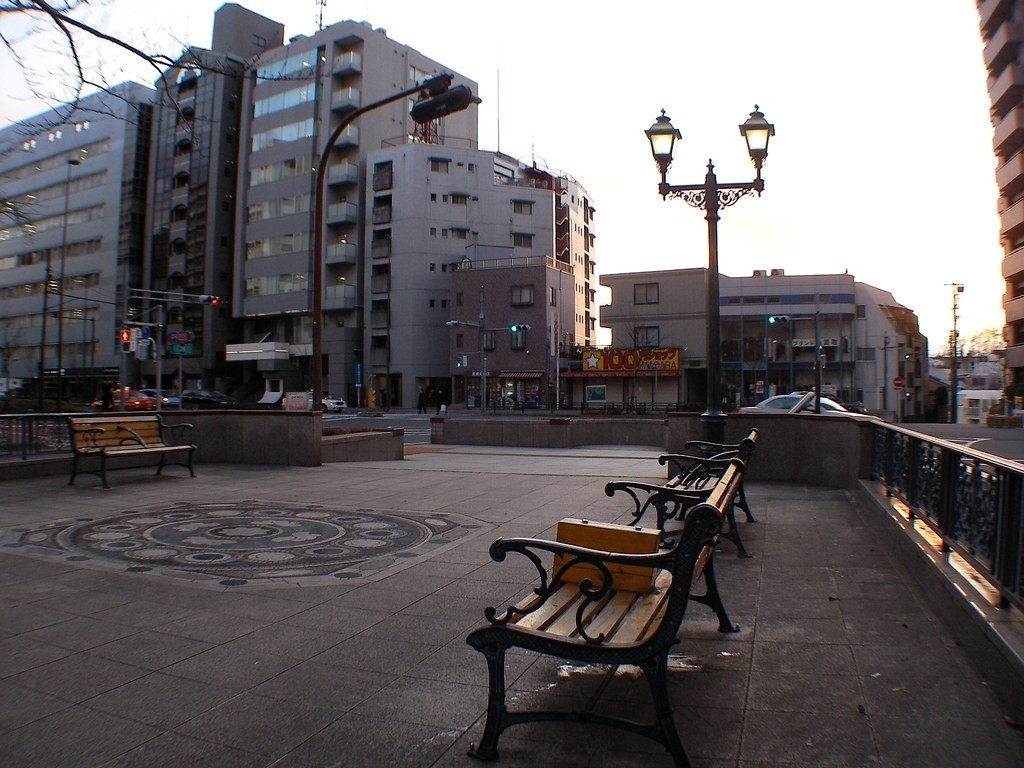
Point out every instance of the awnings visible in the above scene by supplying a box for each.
[499,373,543,378]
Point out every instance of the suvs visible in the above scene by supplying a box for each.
[791,391,868,415]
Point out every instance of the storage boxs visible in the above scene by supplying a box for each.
[552,518,662,593]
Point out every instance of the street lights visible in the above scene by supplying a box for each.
[57,157,80,442]
[556,332,569,409]
[446,320,485,415]
[884,347,901,410]
[643,105,776,466]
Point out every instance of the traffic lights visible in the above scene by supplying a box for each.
[510,325,532,333]
[456,356,462,368]
[767,316,789,326]
[121,330,132,354]
[199,295,223,306]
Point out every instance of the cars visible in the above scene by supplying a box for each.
[282,392,347,414]
[92,389,156,411]
[736,394,883,421]
[140,389,179,410]
[181,389,242,410]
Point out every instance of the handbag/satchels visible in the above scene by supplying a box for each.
[440,405,446,411]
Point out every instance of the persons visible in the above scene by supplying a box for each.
[103,381,119,411]
[418,388,449,415]
[380,389,387,411]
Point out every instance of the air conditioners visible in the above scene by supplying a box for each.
[771,269,784,276]
[754,270,766,277]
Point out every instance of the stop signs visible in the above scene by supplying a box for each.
[894,377,904,388]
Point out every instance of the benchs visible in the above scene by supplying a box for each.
[468,427,760,768]
[65,414,197,489]
